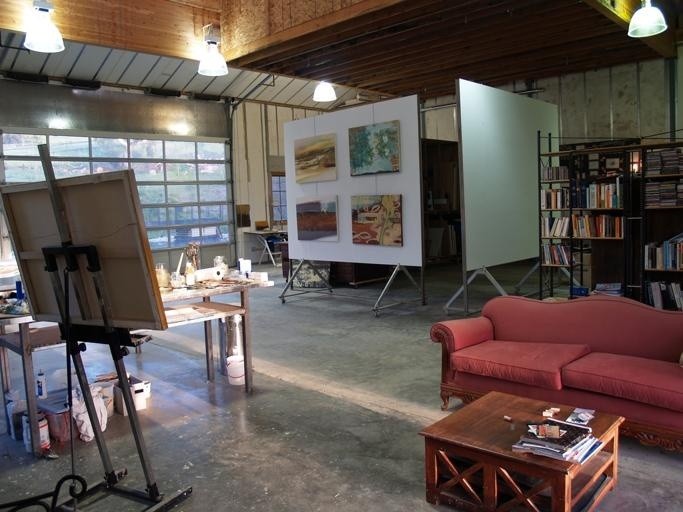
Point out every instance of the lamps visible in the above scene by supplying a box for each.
[312,80,337,103]
[626,1,667,39]
[198,19,229,76]
[22,1,66,54]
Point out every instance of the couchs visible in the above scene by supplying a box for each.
[429,295,683,454]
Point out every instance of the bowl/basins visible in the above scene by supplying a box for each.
[5,299,18,305]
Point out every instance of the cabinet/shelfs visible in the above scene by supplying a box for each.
[536,127,683,310]
[421,137,462,265]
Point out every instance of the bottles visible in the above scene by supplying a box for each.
[185,262,195,285]
[170,272,182,288]
[37,371,48,400]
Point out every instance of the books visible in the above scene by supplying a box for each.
[644,147,682,311]
[540,166,625,298]
[511,408,605,465]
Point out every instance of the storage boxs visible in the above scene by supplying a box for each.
[110,376,151,416]
[37,388,77,441]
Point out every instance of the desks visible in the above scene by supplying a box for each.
[244,229,285,267]
[0,280,274,458]
[418,390,625,512]
